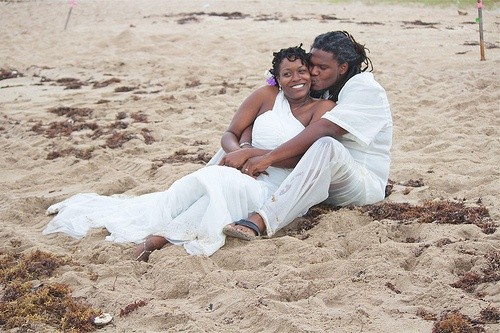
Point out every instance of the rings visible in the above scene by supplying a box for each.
[245,169,249,171]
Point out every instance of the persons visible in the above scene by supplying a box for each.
[206,31,393,240]
[42,43,337,261]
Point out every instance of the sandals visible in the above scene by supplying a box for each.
[136,240,152,262]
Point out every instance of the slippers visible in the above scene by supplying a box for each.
[223,219,269,241]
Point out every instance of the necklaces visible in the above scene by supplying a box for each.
[292,97,308,111]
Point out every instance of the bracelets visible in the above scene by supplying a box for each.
[240,142,250,147]
